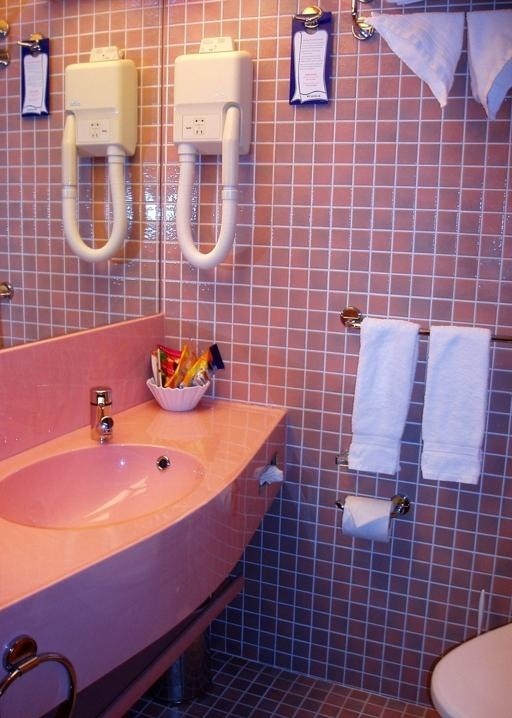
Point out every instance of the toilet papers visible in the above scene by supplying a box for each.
[340,494,396,544]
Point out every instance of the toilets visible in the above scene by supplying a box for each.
[429,623,512,718]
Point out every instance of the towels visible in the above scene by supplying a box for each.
[421,325,491,484]
[363,11,465,109]
[347,316,420,476]
[465,8,512,121]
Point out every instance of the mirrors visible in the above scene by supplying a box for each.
[1,0,165,347]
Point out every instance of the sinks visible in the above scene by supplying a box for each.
[0,439,208,534]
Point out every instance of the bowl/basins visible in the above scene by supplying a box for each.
[146,375,212,412]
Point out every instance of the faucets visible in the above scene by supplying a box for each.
[88,385,115,445]
